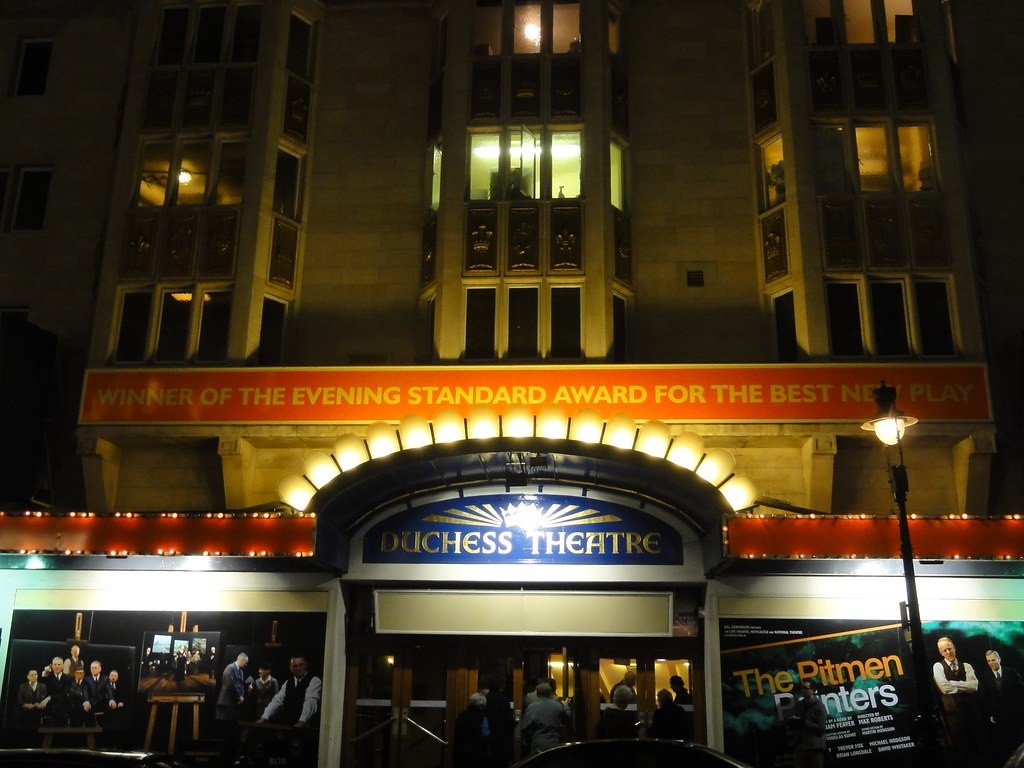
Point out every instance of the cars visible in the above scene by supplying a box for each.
[504,738,750,768]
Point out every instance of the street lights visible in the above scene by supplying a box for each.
[859,379,944,768]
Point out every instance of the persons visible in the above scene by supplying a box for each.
[254,662,279,716]
[522,678,572,753]
[609,670,637,704]
[17,646,124,728]
[256,652,322,729]
[983,650,1024,757]
[487,675,514,768]
[138,644,215,684]
[647,689,689,739]
[670,676,690,704]
[215,652,249,764]
[446,693,492,768]
[591,685,637,738]
[932,637,979,728]
[784,678,827,768]
